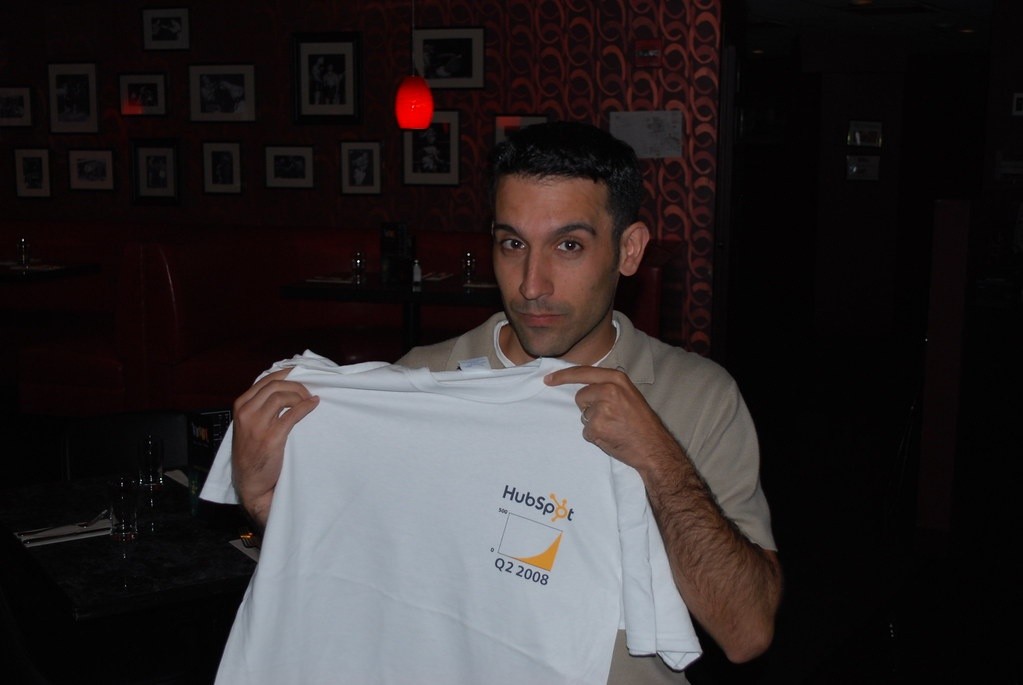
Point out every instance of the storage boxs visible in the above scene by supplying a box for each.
[186,409,231,492]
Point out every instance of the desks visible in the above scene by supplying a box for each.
[283,272,502,357]
[0,490,264,685]
[0,259,103,282]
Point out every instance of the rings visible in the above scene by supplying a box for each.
[580,406,591,425]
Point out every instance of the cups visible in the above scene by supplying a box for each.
[138,431,164,488]
[106,476,140,545]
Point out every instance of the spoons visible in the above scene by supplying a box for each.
[17,508,108,537]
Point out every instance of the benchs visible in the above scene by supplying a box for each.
[0,219,673,418]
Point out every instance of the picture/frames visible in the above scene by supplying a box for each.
[338,139,383,197]
[126,136,180,207]
[0,59,102,135]
[286,31,365,128]
[10,144,116,199]
[409,26,488,91]
[262,144,318,191]
[118,6,258,124]
[492,113,549,148]
[199,140,245,197]
[400,110,462,188]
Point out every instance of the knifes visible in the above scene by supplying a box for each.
[22,527,111,543]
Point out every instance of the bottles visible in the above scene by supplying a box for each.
[411,259,423,282]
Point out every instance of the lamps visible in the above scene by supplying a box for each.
[395,1,435,130]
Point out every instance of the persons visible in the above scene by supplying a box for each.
[232,118,782,685]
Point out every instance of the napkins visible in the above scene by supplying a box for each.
[164,469,189,487]
[13,518,117,548]
[228,538,262,563]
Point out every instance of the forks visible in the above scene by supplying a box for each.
[241,537,261,552]
